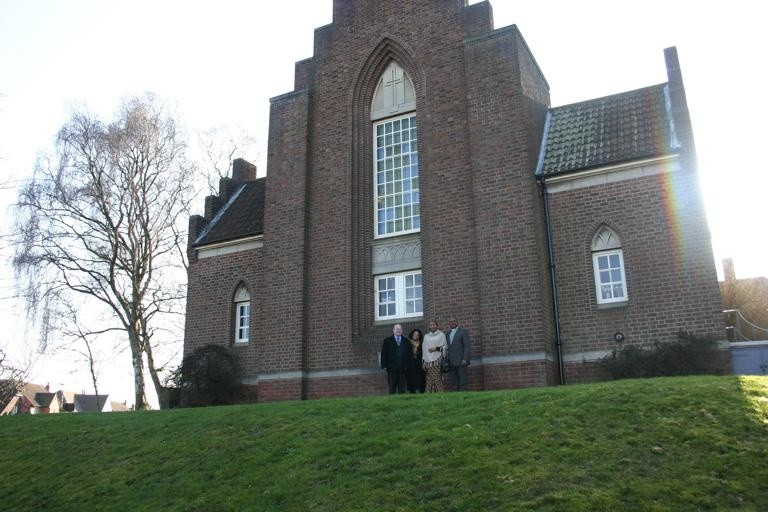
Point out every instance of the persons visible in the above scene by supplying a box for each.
[421,321,448,394]
[407,329,426,393]
[445,318,471,391]
[381,324,413,394]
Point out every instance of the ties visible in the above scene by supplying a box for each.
[397,336,401,346]
[451,329,456,344]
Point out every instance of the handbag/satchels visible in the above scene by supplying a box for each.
[441,347,450,374]
